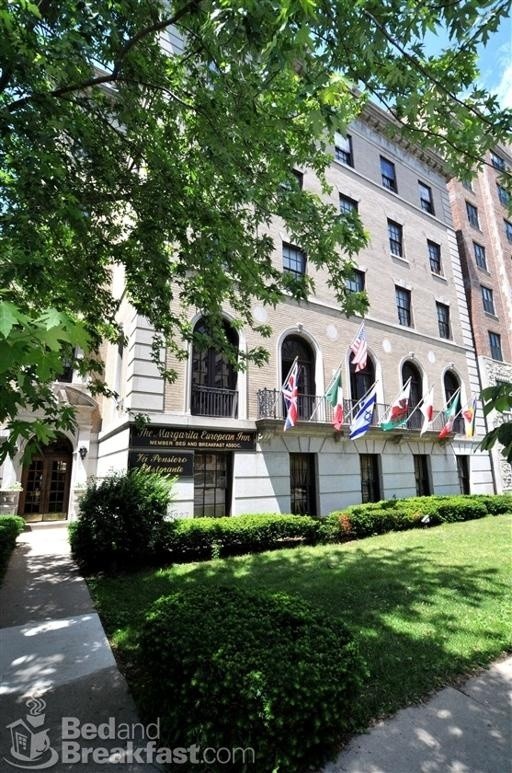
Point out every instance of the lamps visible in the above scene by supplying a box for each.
[79,447,87,461]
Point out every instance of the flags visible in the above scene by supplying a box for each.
[419,388,434,437]
[349,387,376,440]
[379,382,411,431]
[439,392,460,441]
[281,363,299,431]
[325,372,344,432]
[351,323,368,373]
[461,397,477,437]
